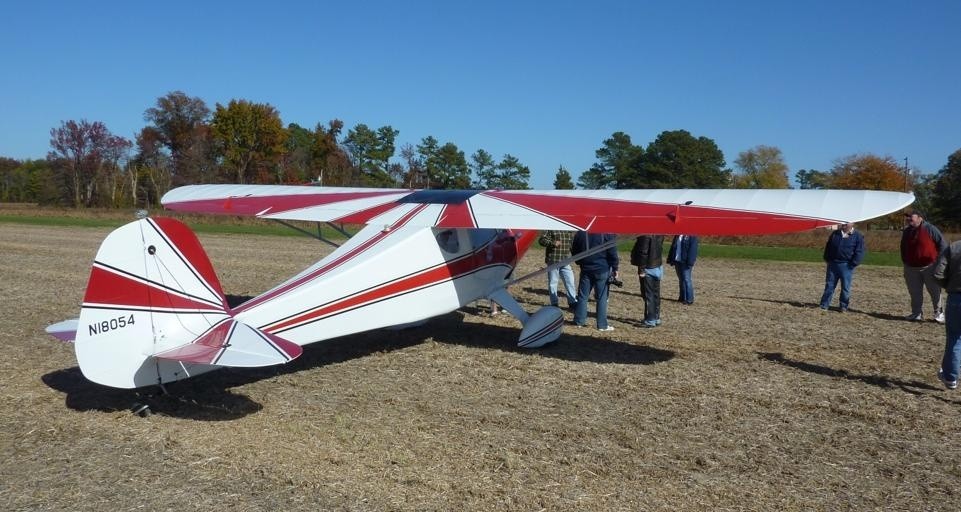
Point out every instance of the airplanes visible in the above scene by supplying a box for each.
[44,179,915,394]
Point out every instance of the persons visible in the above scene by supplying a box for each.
[572,231,620,332]
[630,234,664,328]
[933,240,961,389]
[538,230,578,309]
[818,223,865,313]
[667,235,698,306]
[899,210,948,323]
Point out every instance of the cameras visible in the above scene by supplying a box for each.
[608,271,623,288]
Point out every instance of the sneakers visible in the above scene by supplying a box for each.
[905,313,922,321]
[635,318,661,327]
[934,312,945,322]
[938,368,956,389]
[599,326,614,331]
